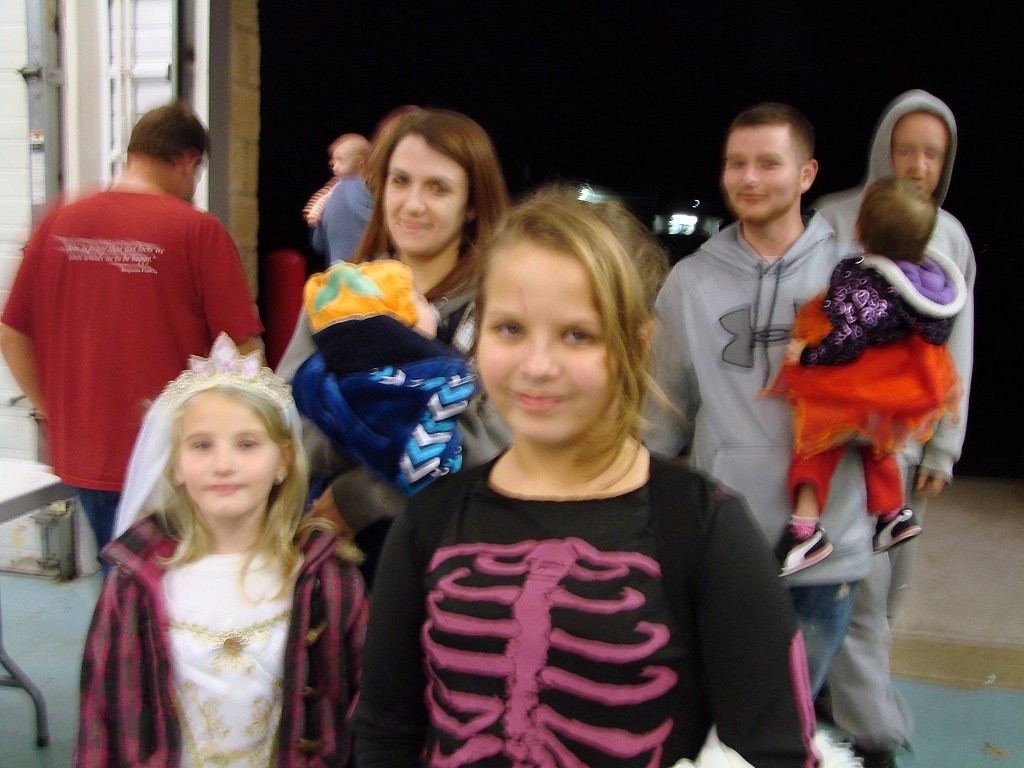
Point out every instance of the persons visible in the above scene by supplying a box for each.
[811,88,977,768]
[71,334,373,768]
[633,103,874,701]
[0,105,269,580]
[267,108,512,597]
[756,176,968,578]
[341,184,864,768]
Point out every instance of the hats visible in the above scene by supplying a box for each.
[303,260,417,332]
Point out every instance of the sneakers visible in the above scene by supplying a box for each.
[771,525,835,579]
[872,508,921,554]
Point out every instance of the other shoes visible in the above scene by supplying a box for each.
[816,685,836,726]
